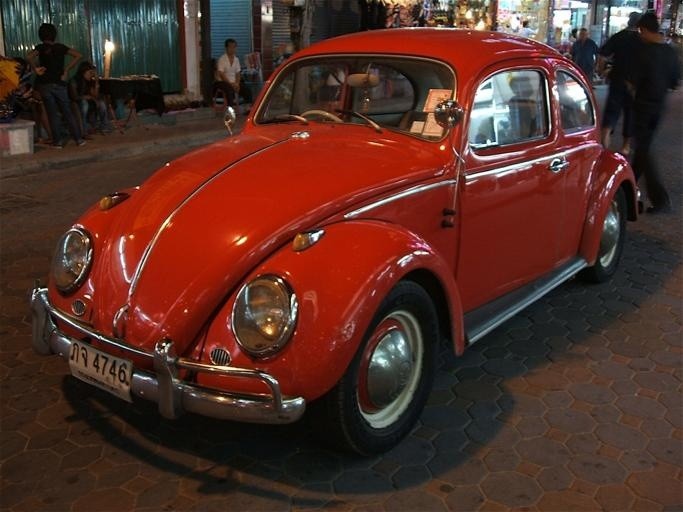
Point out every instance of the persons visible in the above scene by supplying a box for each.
[572,28,601,81]
[592,10,647,156]
[25,22,88,149]
[68,60,111,135]
[518,20,539,38]
[617,12,679,213]
[216,38,257,118]
[8,58,54,145]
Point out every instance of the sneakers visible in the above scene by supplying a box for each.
[84,133,95,142]
[99,128,113,134]
[647,204,672,214]
[50,139,86,149]
[243,110,250,116]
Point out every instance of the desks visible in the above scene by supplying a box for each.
[95,72,163,136]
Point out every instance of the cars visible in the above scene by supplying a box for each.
[29,27,643,459]
